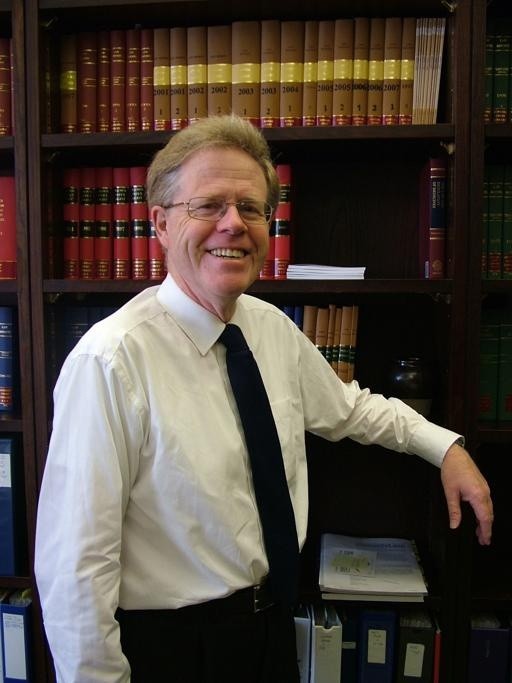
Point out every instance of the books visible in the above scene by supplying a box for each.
[0,1,41,682]
[45,14,445,134]
[46,301,358,441]
[477,35,511,421]
[293,531,512,683]
[43,159,291,279]
[417,158,448,279]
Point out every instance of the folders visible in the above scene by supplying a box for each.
[0,436,25,578]
[292,599,436,683]
[0,586,33,683]
[468,612,512,683]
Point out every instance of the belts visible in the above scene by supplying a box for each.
[225,584,278,612]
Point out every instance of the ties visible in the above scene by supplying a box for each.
[216,323,300,620]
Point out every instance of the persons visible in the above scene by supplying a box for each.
[26,113,496,682]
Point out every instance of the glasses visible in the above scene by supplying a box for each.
[163,196,274,225]
